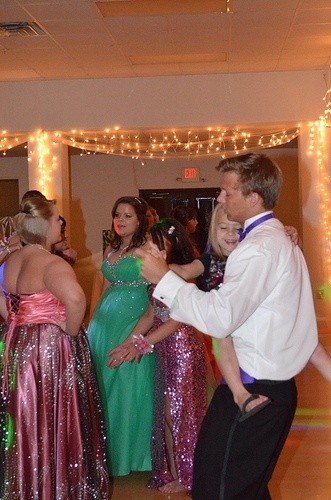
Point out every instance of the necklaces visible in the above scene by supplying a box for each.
[24,243,51,254]
[119,243,131,251]
[237,212,275,242]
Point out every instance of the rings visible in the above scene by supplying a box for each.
[114,359,117,362]
[120,356,124,359]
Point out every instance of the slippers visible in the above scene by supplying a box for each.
[239,394,271,423]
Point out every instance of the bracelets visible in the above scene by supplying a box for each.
[132,334,155,356]
[7,246,12,253]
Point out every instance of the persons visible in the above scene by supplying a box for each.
[85,195,208,495]
[137,152,331,500]
[0,189,113,500]
